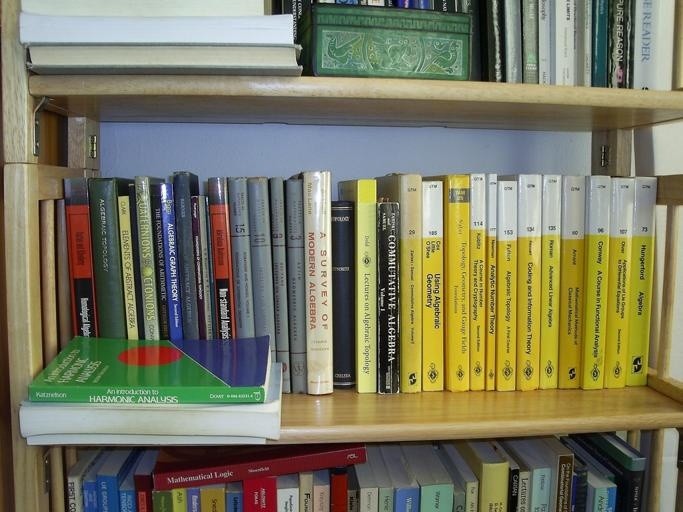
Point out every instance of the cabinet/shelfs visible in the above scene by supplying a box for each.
[0,0,683,512]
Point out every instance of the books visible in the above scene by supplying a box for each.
[485,0,676,91]
[66,429,647,511]
[19,336,283,447]
[20,0,303,80]
[39,169,658,396]
